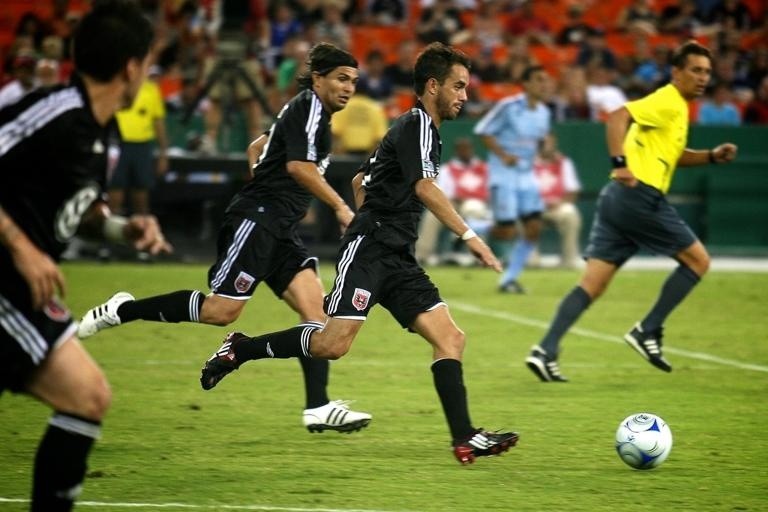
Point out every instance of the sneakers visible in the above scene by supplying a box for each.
[301,398,373,434]
[499,279,527,296]
[199,330,253,393]
[523,343,573,385]
[75,289,138,341]
[451,426,521,467]
[621,320,675,375]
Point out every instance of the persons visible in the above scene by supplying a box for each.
[200,41,521,465]
[523,43,739,383]
[470,61,557,294]
[75,43,373,434]
[0,4,175,509]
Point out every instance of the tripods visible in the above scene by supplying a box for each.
[180,58,277,142]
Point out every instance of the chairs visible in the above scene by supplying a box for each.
[0,2,768,148]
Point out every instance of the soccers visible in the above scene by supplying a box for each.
[615,413,673,470]
